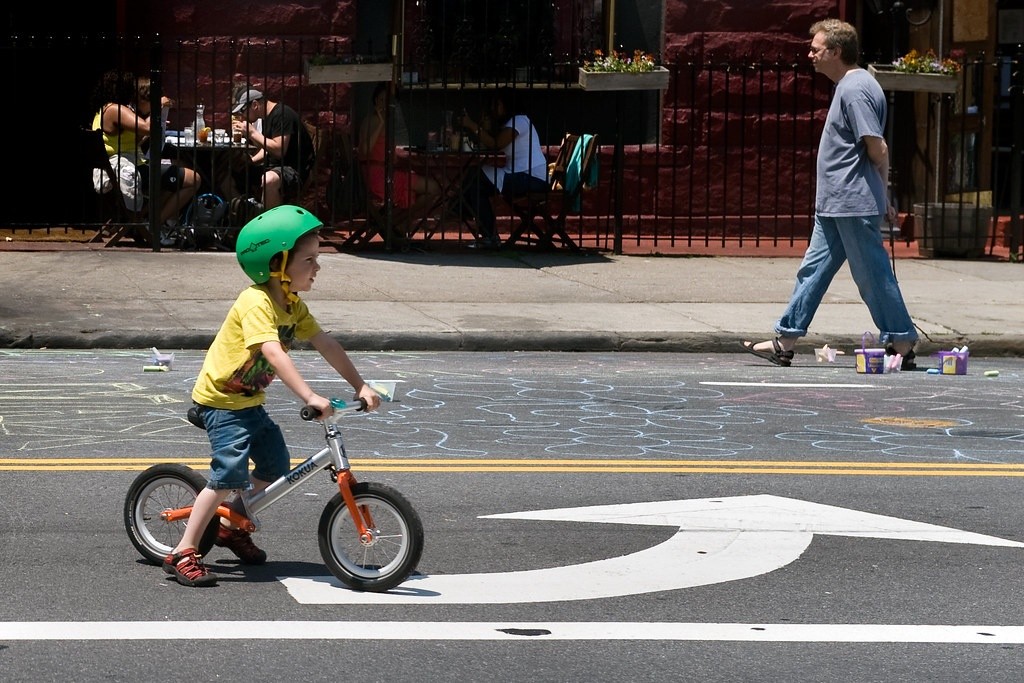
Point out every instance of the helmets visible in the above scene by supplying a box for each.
[236,205,323,285]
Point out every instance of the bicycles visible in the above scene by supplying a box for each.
[124,397,425,594]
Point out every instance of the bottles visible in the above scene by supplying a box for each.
[192,105,205,131]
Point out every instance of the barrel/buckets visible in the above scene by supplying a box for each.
[854,331,885,374]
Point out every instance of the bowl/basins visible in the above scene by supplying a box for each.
[151,354,174,370]
[939,351,968,375]
[814,349,837,363]
[913,202,993,259]
[367,382,396,401]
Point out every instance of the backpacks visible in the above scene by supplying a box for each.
[185,193,263,251]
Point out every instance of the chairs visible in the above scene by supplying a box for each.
[502,132,601,255]
[77,125,162,248]
[293,120,326,205]
[332,132,433,250]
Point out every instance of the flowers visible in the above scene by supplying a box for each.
[891,48,963,77]
[583,49,655,73]
[312,49,385,66]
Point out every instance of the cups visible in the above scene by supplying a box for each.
[197,129,208,141]
[233,129,242,145]
[429,133,474,153]
[184,127,197,146]
[215,129,225,143]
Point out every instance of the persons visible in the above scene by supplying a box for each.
[357,83,452,227]
[219,85,316,211]
[162,205,382,588]
[445,86,551,250]
[92,67,201,245]
[738,18,918,370]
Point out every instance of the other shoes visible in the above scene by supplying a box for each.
[145,220,175,245]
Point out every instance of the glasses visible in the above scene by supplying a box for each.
[240,107,249,114]
[807,45,835,56]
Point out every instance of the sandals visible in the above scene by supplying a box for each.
[739,336,794,367]
[163,548,218,587]
[215,521,266,563]
[886,346,916,369]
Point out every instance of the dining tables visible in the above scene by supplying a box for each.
[402,147,509,248]
[142,128,259,247]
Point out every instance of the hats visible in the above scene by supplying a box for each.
[232,90,263,113]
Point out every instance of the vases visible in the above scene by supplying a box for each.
[579,66,670,91]
[303,54,393,84]
[868,64,960,92]
[913,202,993,258]
